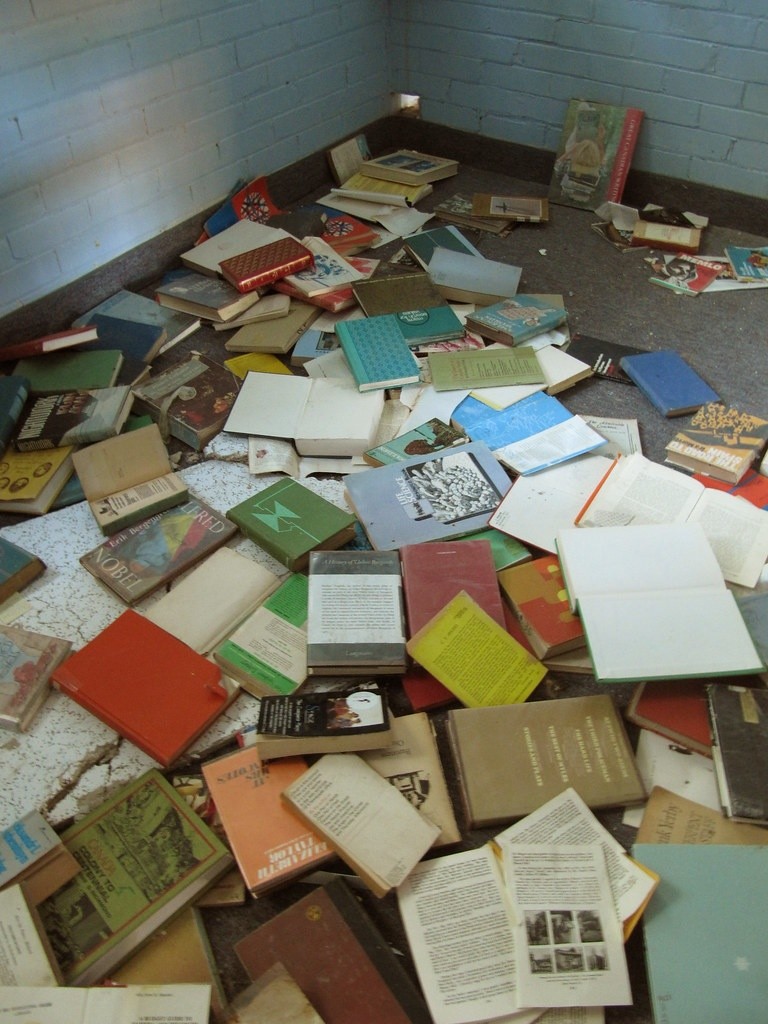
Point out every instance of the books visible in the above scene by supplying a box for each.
[548,98,644,212]
[0,132,768,1024]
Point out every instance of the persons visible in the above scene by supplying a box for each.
[0,462,9,475]
[327,719,360,729]
[9,478,28,492]
[295,264,330,280]
[325,260,336,267]
[330,266,342,275]
[314,256,329,265]
[333,712,358,721]
[33,462,52,478]
[0,477,10,489]
[652,258,699,290]
